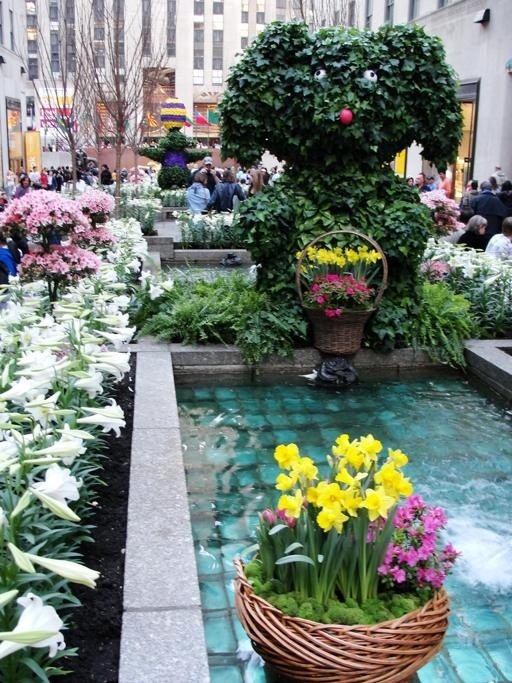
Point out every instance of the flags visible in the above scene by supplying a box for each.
[51,106,222,133]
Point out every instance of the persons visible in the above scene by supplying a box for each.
[186,156,280,216]
[409,164,512,266]
[0,149,129,293]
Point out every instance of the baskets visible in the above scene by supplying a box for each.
[295,230,389,356]
[232,552,451,682]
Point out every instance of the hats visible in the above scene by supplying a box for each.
[203,156,213,165]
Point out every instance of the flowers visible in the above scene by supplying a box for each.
[294,244,385,322]
[241,432,463,625]
